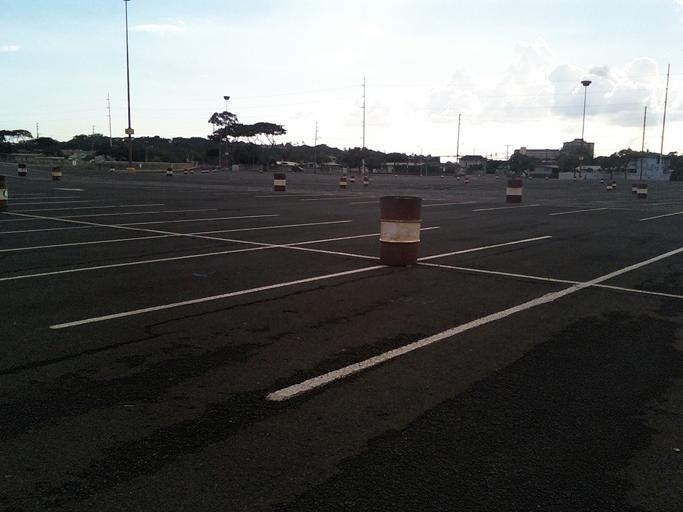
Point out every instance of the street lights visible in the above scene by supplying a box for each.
[224,96,230,111]
[581,81,592,139]
